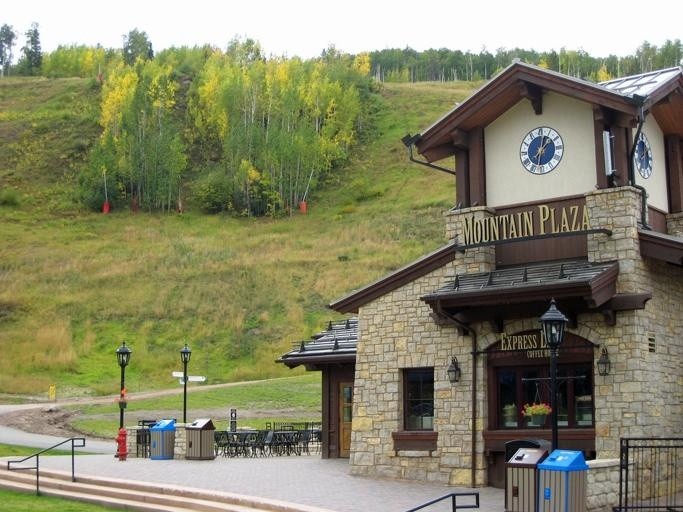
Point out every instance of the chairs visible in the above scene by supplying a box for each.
[214,426,322,458]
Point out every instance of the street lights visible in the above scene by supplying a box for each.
[178,343,191,422]
[540,299,569,454]
[114,340,132,456]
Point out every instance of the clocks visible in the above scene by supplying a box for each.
[634,132,652,179]
[520,126,564,175]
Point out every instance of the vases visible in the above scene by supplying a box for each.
[531,415,546,425]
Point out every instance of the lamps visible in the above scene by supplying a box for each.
[447,357,461,384]
[597,347,611,376]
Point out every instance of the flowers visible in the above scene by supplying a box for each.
[521,402,552,417]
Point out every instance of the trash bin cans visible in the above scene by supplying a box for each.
[506,449,589,512]
[151,420,176,460]
[186,418,214,460]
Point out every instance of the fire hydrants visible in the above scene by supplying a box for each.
[114,428,127,460]
